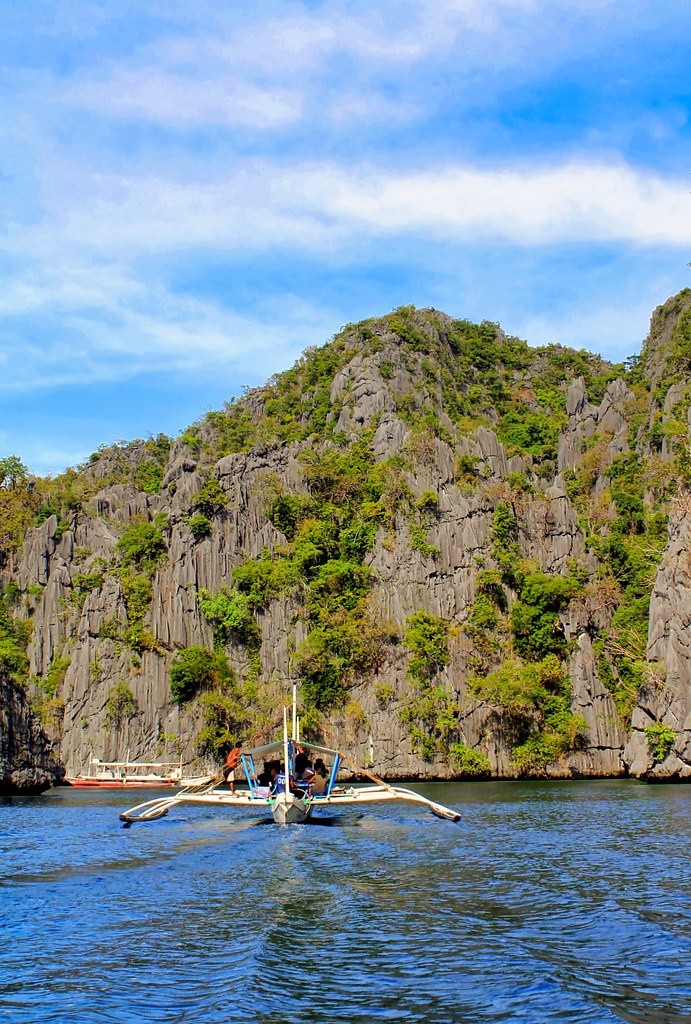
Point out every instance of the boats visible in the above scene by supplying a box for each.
[63,747,215,789]
[118,683,462,829]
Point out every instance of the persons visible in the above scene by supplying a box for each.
[223,741,242,796]
[250,734,330,797]
